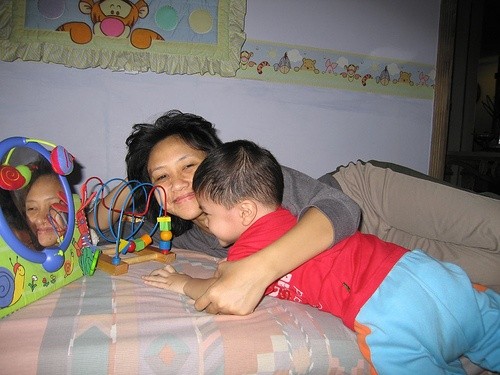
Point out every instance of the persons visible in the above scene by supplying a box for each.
[142,139,500,374]
[2,151,83,253]
[86,108,500,315]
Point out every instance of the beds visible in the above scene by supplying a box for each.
[0,243,494,375]
